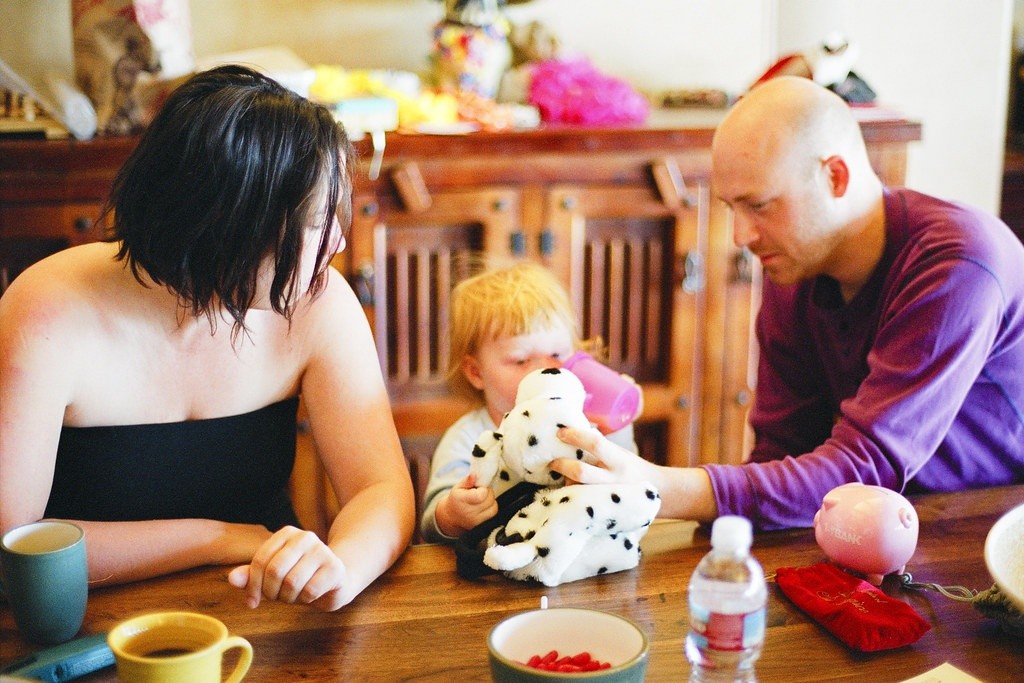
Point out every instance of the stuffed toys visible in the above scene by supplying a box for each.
[463,366,663,585]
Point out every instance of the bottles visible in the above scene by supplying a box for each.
[506,368,593,485]
[683,514,769,683]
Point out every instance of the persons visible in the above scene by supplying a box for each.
[416,258,638,542]
[0,66,414,612]
[549,74,1024,530]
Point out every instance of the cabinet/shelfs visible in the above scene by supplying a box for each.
[0,105,921,470]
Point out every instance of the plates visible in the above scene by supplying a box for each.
[985,503,1024,618]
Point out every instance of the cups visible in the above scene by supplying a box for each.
[107,610,253,683]
[561,351,640,431]
[484,606,652,683]
[1,520,88,645]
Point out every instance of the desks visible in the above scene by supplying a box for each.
[0,483,1024,683]
